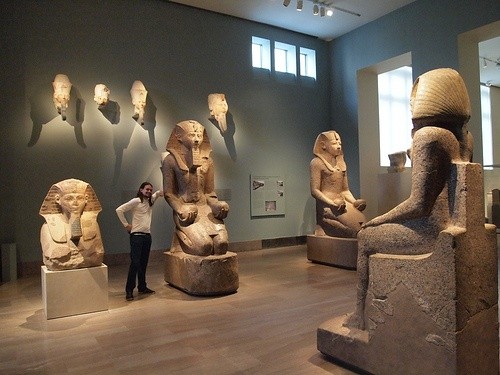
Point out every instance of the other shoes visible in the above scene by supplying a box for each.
[126,293,134,301]
[138,287,155,294]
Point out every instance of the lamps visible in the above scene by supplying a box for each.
[283,0,333,16]
[483,57,500,87]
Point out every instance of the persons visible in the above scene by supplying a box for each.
[115,182,165,300]
[310,131,366,239]
[342,68,474,331]
[38,179,104,271]
[162,121,229,256]
